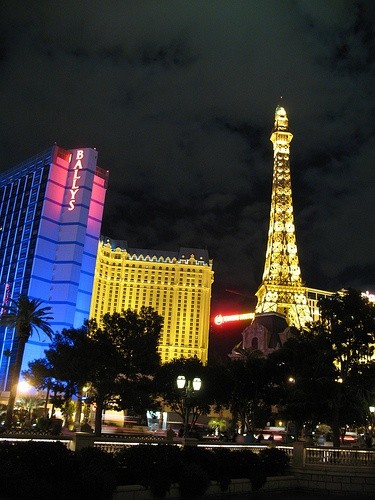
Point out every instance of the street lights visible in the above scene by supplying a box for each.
[176,376,202,437]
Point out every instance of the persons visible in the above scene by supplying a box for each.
[214,425,220,437]
[325,432,333,442]
[166,425,176,437]
[258,434,264,441]
[80,419,91,432]
[178,424,184,437]
[269,432,274,440]
